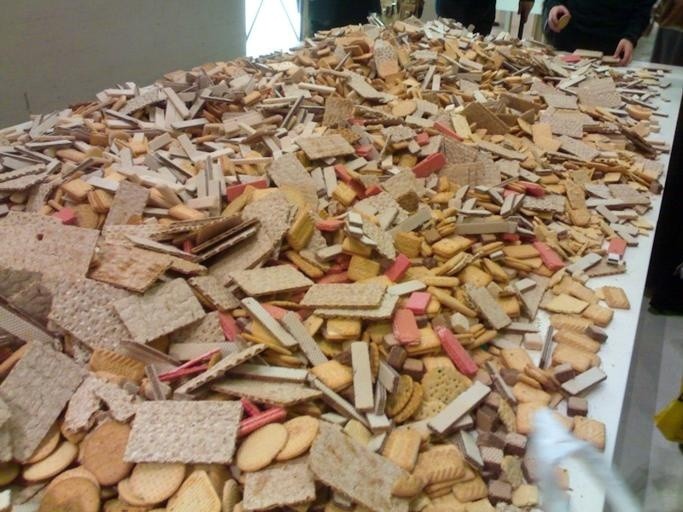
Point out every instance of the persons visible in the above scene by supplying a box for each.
[540,0,655,65]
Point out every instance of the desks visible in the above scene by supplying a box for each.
[523,48,683,512]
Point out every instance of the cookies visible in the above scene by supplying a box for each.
[0,14,673,512]
